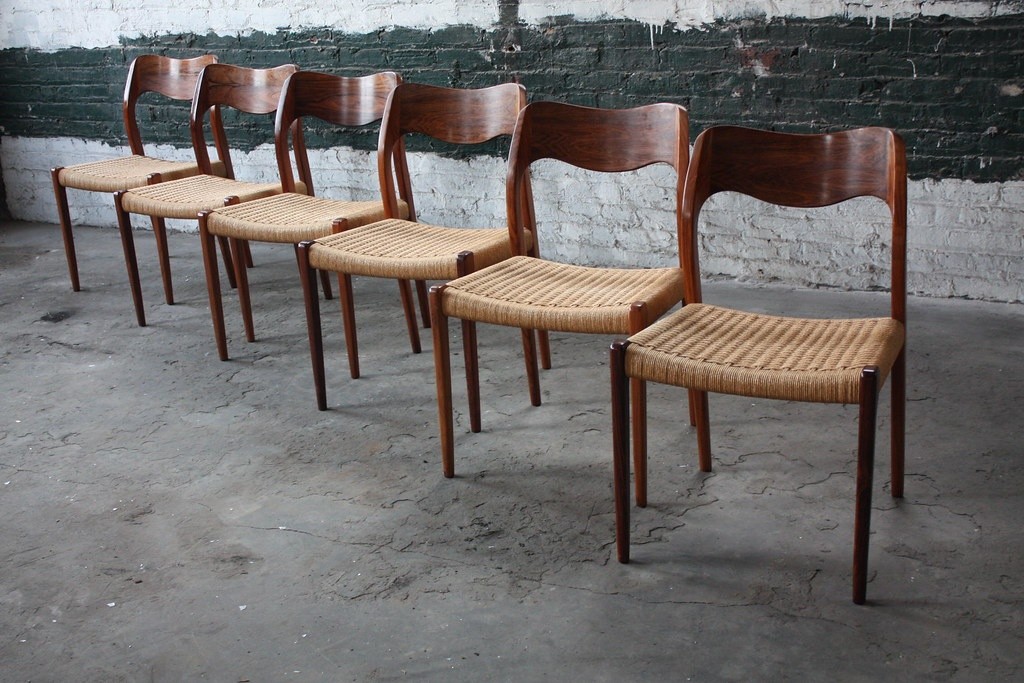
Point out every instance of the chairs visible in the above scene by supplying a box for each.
[49,55,254,304]
[198,72,433,379]
[609,124,907,604]
[296,85,553,434]
[113,63,334,346]
[426,100,700,508]
